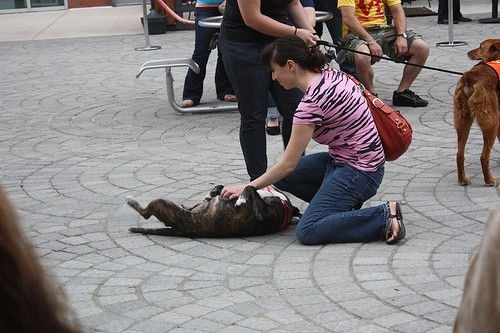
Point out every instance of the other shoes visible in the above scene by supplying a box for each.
[219,94,236,101]
[179,99,200,107]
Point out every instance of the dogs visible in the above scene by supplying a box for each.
[125,184,303,238]
[453,39,500,186]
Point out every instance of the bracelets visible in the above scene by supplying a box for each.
[294,27,298,34]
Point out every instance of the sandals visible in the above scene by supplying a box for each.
[387,202,406,245]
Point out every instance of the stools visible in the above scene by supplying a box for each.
[135,58,237,111]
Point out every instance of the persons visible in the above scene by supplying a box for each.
[220,35,407,245]
[177,0,428,182]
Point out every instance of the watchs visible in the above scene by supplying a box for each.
[396,33,407,39]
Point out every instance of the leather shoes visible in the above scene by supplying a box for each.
[267,119,280,135]
[437,17,472,24]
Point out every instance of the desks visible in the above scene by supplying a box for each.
[198,11,333,28]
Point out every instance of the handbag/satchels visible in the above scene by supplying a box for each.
[340,70,413,161]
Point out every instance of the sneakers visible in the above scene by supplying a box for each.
[392,88,428,107]
[370,89,379,98]
